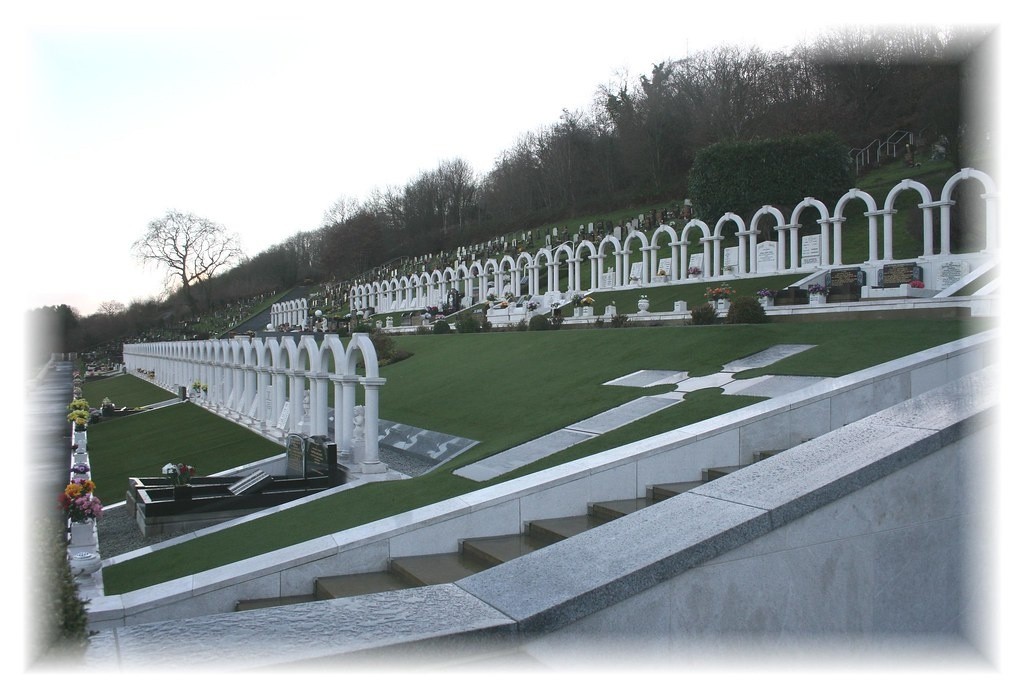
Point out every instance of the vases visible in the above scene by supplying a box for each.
[74,431,86,447]
[582,307,593,317]
[707,301,718,310]
[174,483,194,505]
[71,519,99,546]
[757,296,775,307]
[527,311,538,319]
[810,291,826,305]
[195,392,201,396]
[718,299,732,311]
[422,320,430,326]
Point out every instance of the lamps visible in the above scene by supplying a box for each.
[315,308,323,325]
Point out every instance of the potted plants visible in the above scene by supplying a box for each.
[102,397,113,417]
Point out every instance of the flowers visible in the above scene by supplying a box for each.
[162,463,196,485]
[757,288,779,297]
[580,297,595,307]
[67,400,89,431]
[192,380,201,393]
[526,301,540,312]
[808,283,829,296]
[420,313,431,320]
[56,479,104,523]
[704,281,737,303]
